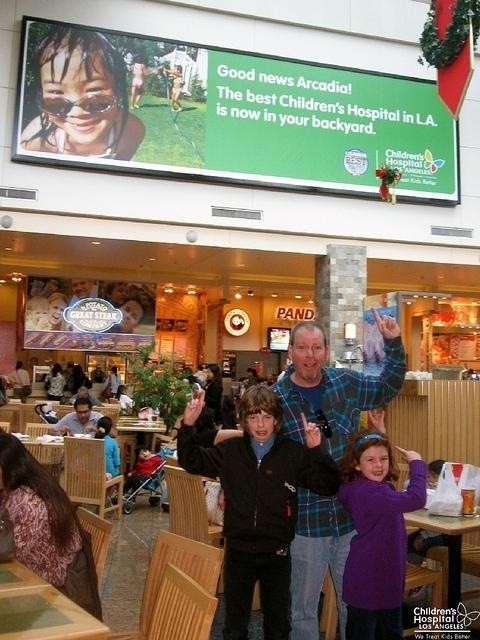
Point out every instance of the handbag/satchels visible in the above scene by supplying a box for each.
[45,381,51,390]
[205,481,225,527]
[101,384,112,398]
[23,385,31,395]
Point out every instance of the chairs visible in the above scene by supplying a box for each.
[142,456,479,639]
[1,385,162,640]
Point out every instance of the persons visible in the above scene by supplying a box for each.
[177,386,341,639]
[129,54,157,107]
[26,277,154,334]
[21,25,146,162]
[0,427,103,623]
[163,65,185,112]
[0,356,259,506]
[265,305,405,640]
[337,409,426,639]
[404,459,448,566]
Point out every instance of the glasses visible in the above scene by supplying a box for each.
[316,409,332,438]
[36,92,118,115]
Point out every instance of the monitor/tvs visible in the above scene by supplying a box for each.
[267,326,292,353]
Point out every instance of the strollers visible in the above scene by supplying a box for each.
[35,403,59,424]
[111,427,180,514]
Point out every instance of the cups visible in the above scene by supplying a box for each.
[151,415,158,421]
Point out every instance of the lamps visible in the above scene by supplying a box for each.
[344,322,358,346]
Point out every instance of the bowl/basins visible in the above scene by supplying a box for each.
[73,433,92,438]
